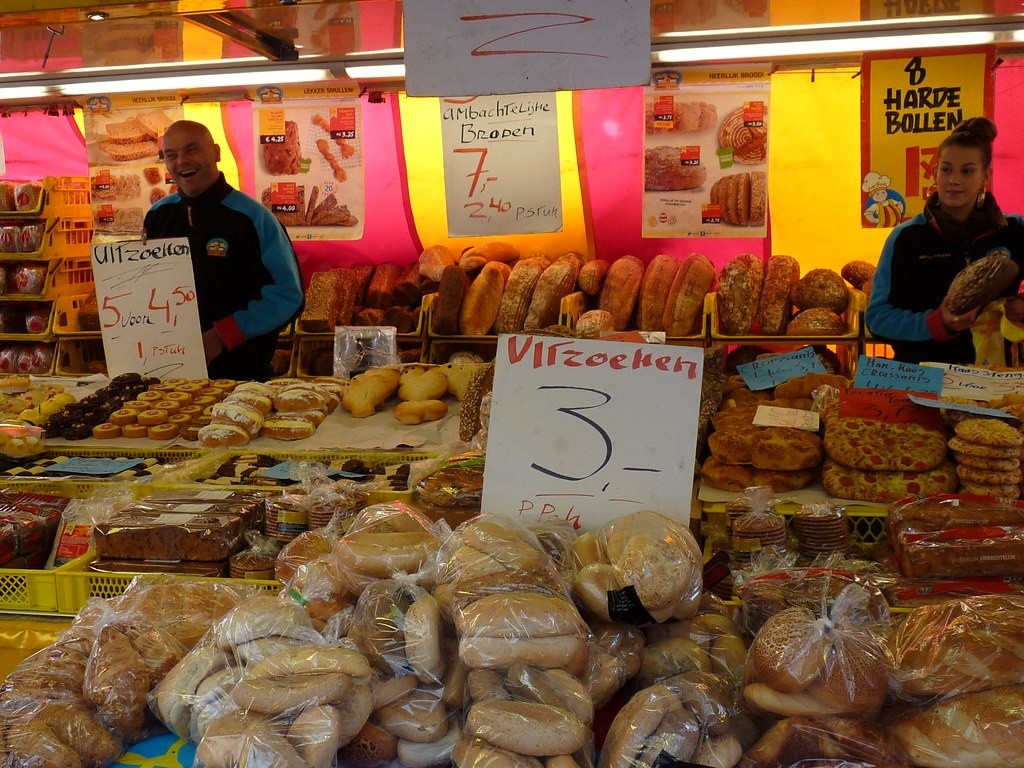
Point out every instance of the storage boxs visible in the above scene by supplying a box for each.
[0,176,886,613]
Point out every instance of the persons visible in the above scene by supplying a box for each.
[143,121,305,382]
[864,117,1024,365]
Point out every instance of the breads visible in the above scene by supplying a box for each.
[1,180,1024,768]
[645,145,706,190]
[98,109,173,161]
[264,121,302,177]
[710,170,766,227]
[261,185,358,227]
[646,102,718,134]
[719,104,769,163]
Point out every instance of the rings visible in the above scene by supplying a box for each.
[953,316,959,322]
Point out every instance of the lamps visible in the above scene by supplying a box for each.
[0,11,1024,100]
